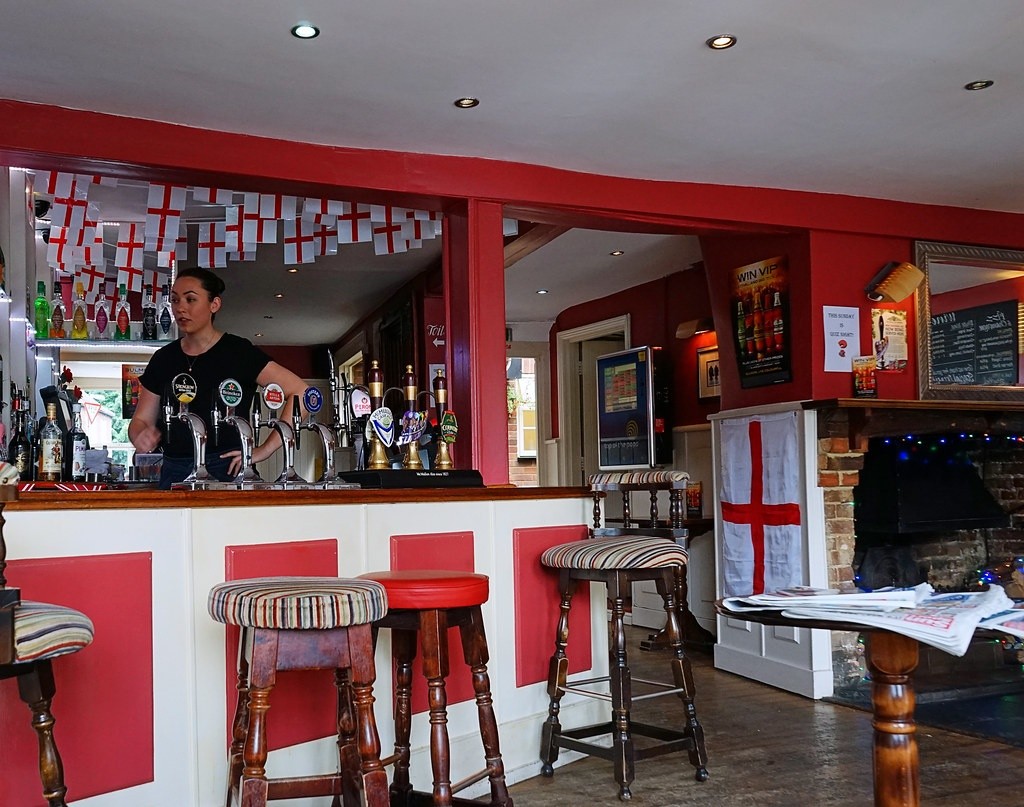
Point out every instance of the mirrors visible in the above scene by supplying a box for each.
[914,241,1024,404]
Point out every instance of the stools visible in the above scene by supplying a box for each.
[539,471,709,802]
[1,482,94,806]
[207,576,391,807]
[331,570,515,807]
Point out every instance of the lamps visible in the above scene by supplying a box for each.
[675,317,714,339]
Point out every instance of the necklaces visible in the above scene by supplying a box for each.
[181,331,216,371]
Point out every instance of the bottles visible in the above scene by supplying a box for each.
[115,284,130,340]
[70,283,88,340]
[157,284,174,341]
[737,291,783,358]
[49,281,66,339]
[94,283,110,340]
[0,393,86,482]
[142,284,157,340]
[33,280,49,339]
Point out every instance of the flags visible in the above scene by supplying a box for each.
[33,169,443,307]
[719,411,802,598]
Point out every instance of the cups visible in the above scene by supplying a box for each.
[129,464,162,482]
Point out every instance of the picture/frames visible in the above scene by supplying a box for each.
[697,345,721,403]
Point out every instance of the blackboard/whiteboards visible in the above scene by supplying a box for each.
[930,297,1018,386]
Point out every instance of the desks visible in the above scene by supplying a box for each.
[715,595,1024,807]
[605,515,715,652]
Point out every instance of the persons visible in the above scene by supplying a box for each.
[128,267,310,490]
